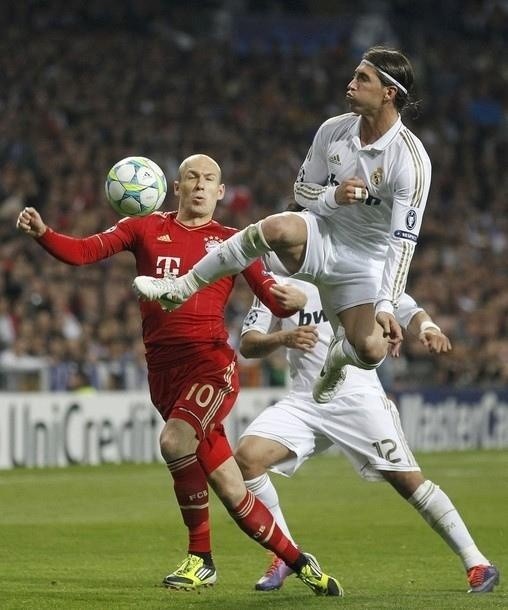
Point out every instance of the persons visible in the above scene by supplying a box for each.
[13,153,346,598]
[130,45,432,405]
[0,1,508,395]
[232,202,501,594]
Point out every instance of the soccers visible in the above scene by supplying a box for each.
[105,157,167,217]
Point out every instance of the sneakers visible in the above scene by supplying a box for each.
[299,552,343,596]
[132,276,188,311]
[467,561,499,594]
[164,553,217,590]
[313,336,347,403]
[256,555,293,590]
